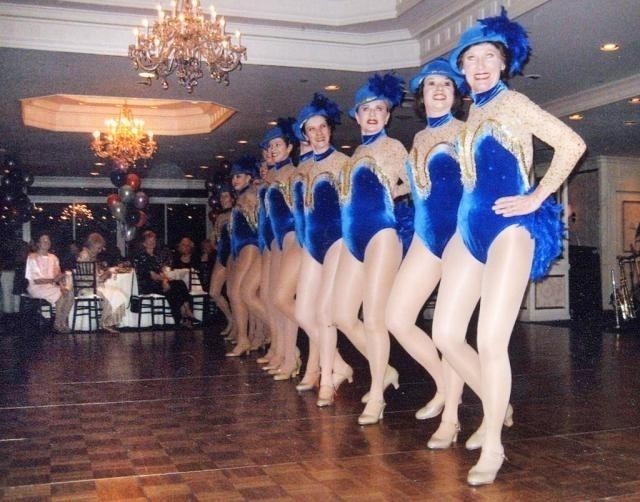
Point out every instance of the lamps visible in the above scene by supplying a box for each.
[90,98,159,172]
[124,1,248,95]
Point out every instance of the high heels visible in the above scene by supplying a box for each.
[357,399,389,426]
[360,363,400,404]
[254,344,303,382]
[465,403,516,450]
[218,326,273,358]
[295,363,355,408]
[414,386,464,420]
[426,420,461,451]
[466,443,507,487]
[100,321,122,336]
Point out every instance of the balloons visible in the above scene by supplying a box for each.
[0,155,33,231]
[205,160,234,224]
[107,158,149,241]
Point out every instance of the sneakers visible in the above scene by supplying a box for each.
[176,313,204,332]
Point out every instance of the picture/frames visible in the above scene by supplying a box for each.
[620,199,640,254]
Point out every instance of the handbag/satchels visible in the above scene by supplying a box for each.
[129,295,143,314]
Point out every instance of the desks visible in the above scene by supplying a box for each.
[63,264,204,335]
[0,271,22,312]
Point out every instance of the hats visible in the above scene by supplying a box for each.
[256,125,294,152]
[448,22,520,79]
[407,55,472,100]
[291,103,331,143]
[347,81,398,122]
[214,160,268,201]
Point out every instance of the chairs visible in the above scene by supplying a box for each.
[68,260,105,340]
[15,264,70,342]
[136,267,174,333]
[183,264,221,328]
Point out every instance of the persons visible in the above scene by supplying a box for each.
[292,92,354,406]
[208,117,314,381]
[25,230,218,334]
[386,57,471,449]
[431,4,587,486]
[331,71,410,424]
